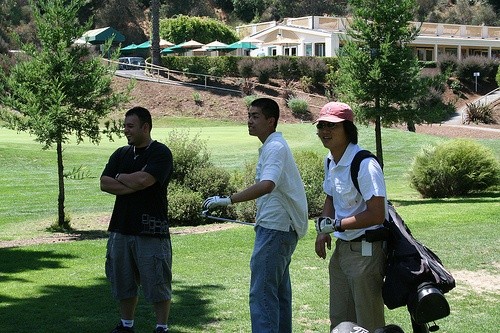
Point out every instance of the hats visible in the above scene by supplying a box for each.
[312,101,354,124]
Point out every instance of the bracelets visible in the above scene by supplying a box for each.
[116,173,120,178]
[336,218,345,232]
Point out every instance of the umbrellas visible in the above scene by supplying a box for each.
[160,36,264,56]
[121,40,152,58]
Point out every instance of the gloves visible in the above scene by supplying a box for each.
[202,195,233,209]
[314,216,345,234]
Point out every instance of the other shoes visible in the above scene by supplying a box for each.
[109,320,135,333]
[153,326,168,333]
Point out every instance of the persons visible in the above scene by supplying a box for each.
[201,97,309,333]
[99,107,173,333]
[314,102,389,333]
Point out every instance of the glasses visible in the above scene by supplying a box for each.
[316,121,342,129]
